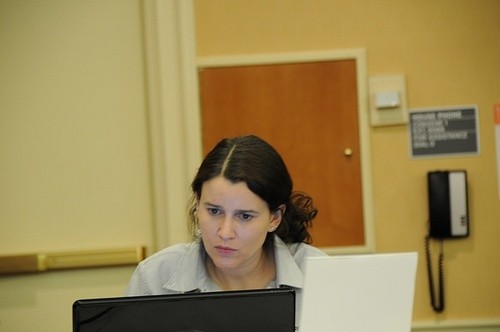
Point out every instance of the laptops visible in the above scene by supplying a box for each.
[72,288,296,332]
[299,252,417,332]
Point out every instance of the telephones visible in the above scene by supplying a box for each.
[428,169,469,240]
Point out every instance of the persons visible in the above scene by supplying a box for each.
[125,135,329,331]
